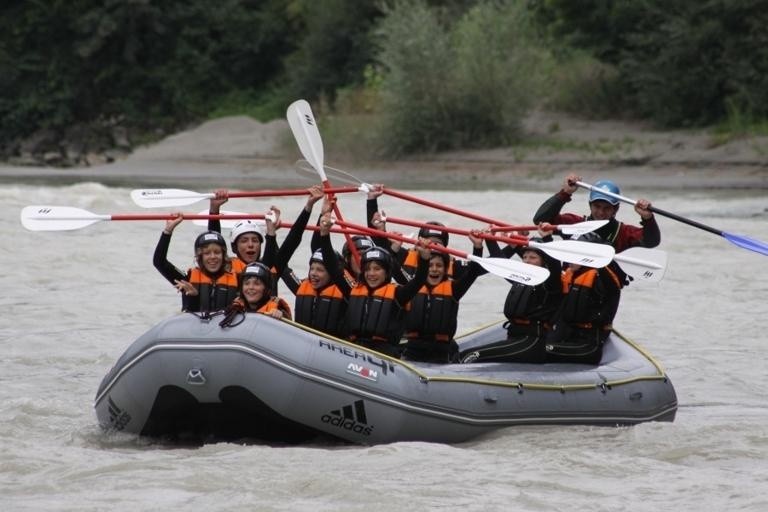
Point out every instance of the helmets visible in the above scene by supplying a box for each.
[231,220,264,253]
[240,262,273,285]
[342,236,375,255]
[195,231,227,254]
[418,221,449,248]
[309,248,344,274]
[361,248,394,269]
[520,238,546,257]
[571,231,602,243]
[417,241,450,268]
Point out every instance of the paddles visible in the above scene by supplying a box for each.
[567,179,768,257]
[509,233,668,283]
[490,219,610,236]
[322,217,551,286]
[131,183,369,209]
[193,211,375,237]
[20,204,278,231]
[286,98,361,271]
[374,209,616,269]
[295,158,515,227]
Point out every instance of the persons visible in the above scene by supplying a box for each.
[207,184,326,298]
[262,207,353,342]
[500,228,619,365]
[532,173,660,254]
[372,211,486,367]
[224,260,295,322]
[456,221,565,365]
[152,212,245,315]
[310,195,406,302]
[364,183,526,288]
[318,212,433,359]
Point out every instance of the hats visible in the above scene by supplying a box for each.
[589,180,621,205]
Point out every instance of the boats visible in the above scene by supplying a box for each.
[94,310,678,449]
